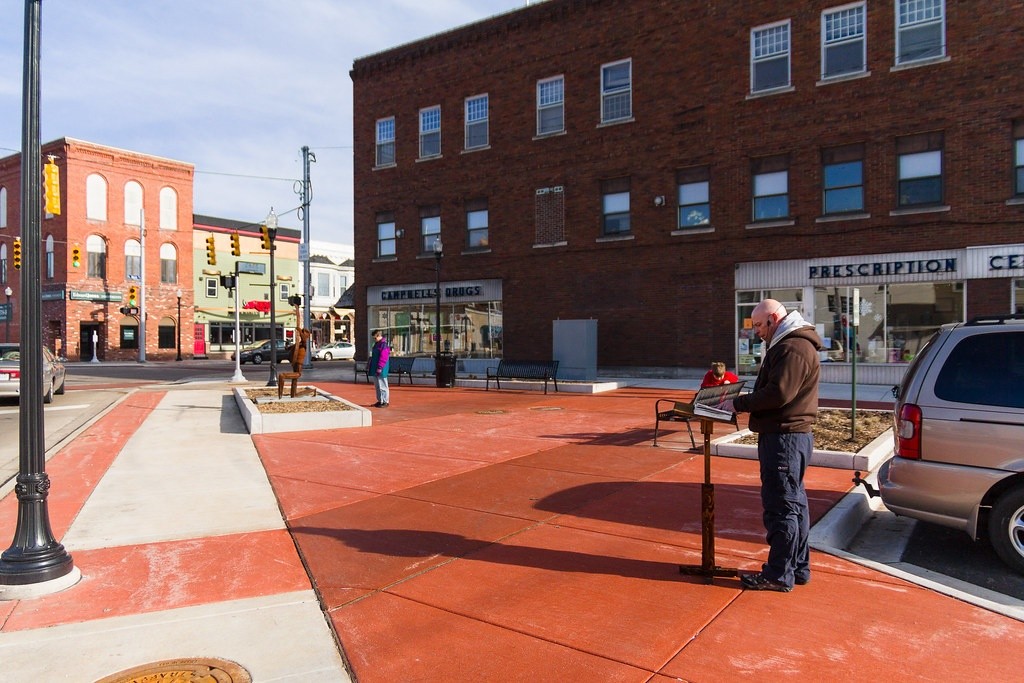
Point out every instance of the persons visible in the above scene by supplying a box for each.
[366,330,390,408]
[700,362,739,389]
[717,299,822,592]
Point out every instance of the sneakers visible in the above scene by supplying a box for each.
[741,573,793,591]
[762,563,809,584]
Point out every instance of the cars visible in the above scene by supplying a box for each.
[312,342,356,361]
[817,338,844,361]
[231,338,293,365]
[0,342,66,404]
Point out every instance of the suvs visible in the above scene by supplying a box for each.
[875,313,1024,578]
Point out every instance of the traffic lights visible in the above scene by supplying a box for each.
[41,163,60,215]
[13,242,21,269]
[72,247,80,267]
[220,276,234,289]
[259,225,270,250]
[205,238,216,266]
[129,308,138,315]
[230,234,241,256]
[128,287,138,306]
[120,307,127,314]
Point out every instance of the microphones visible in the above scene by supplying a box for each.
[764,325,769,340]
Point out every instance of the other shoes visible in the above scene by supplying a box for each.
[379,403,388,407]
[374,401,381,406]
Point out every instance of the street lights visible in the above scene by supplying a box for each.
[175,289,183,361]
[266,208,280,387]
[432,235,442,385]
[4,286,12,342]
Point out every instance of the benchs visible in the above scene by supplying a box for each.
[353,356,416,386]
[652,380,748,450]
[486,359,559,395]
